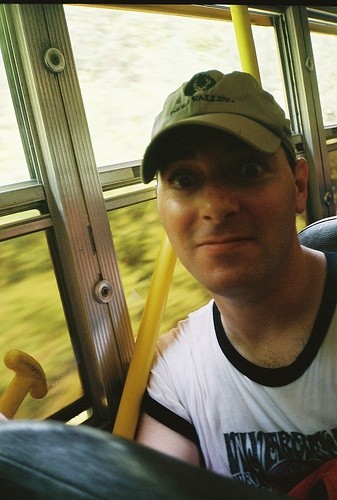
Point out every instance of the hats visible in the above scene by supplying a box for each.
[141,70,295,184]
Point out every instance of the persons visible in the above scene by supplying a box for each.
[134,70,337,500]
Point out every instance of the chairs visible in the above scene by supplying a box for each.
[0,215,337,500]
